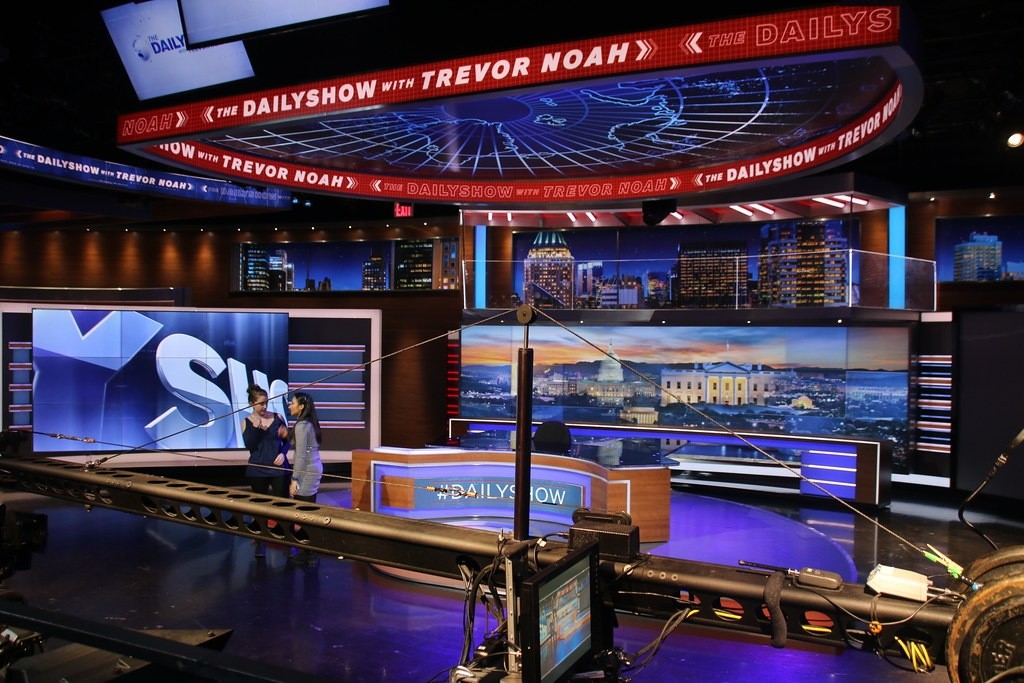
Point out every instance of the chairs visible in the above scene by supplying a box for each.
[533,422,572,456]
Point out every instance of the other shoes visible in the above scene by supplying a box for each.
[292,551,318,564]
[255,546,265,557]
[284,550,294,558]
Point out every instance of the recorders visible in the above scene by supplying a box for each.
[566,507,639,563]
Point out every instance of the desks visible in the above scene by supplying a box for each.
[352,449,671,542]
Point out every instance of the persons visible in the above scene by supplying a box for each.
[287,393,324,566]
[243,384,292,559]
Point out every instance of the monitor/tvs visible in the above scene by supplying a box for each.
[520,539,603,683]
